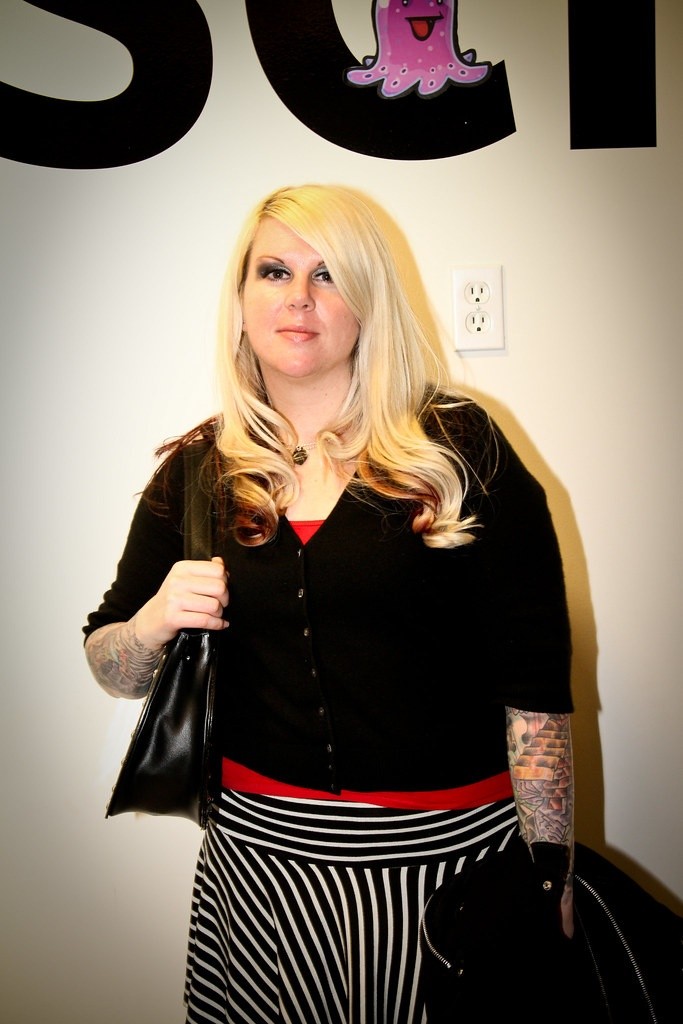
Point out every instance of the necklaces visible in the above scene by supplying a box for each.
[292,442,316,465]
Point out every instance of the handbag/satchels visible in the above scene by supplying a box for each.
[106,440,222,828]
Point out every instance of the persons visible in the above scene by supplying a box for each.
[81,185,575,1024]
